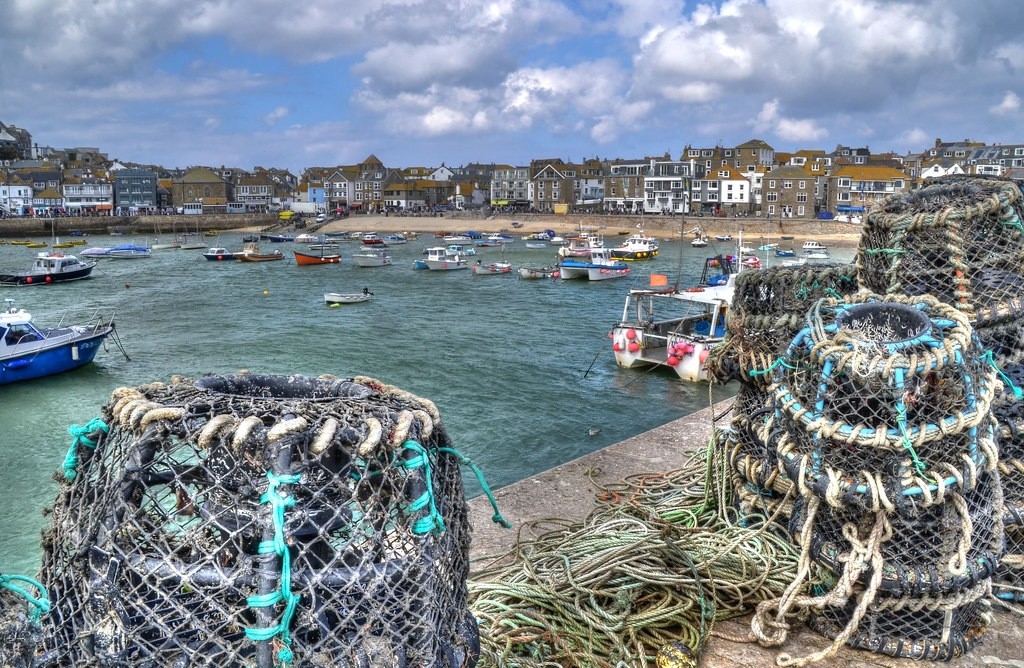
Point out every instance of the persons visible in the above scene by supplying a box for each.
[498,204,696,217]
[22,206,178,218]
[710,203,716,221]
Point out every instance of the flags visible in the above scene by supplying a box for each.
[780,176,784,195]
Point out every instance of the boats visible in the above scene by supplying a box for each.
[202,247,244,261]
[608,174,775,385]
[152,219,832,282]
[475,261,513,275]
[519,266,561,279]
[352,252,393,268]
[233,241,287,262]
[323,284,375,306]
[293,233,342,266]
[105,235,152,259]
[79,247,113,258]
[689,235,709,247]
[0,296,116,385]
[422,247,469,271]
[0,251,97,288]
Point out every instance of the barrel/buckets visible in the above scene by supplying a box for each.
[714,326,725,337]
[694,321,711,336]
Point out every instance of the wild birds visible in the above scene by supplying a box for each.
[586,429,603,436]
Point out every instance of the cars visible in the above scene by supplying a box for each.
[315,214,327,223]
